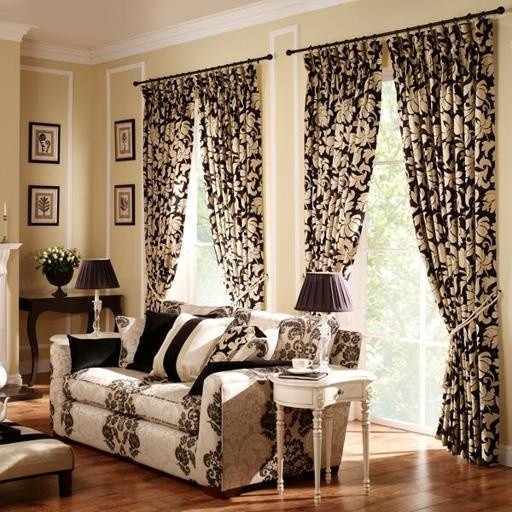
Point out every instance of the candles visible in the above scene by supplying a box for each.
[3,200,7,215]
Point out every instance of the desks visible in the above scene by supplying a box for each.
[19,295,125,387]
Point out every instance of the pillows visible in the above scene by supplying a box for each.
[66,309,293,396]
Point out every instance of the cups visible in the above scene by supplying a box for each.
[287,356,317,369]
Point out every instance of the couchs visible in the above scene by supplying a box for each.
[50,299,362,499]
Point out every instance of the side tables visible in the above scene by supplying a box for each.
[269,370,377,495]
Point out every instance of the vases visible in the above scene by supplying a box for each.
[46,272,73,297]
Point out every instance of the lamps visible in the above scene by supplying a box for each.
[73,257,121,337]
[294,272,355,372]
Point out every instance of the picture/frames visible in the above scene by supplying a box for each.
[114,119,135,161]
[114,183,135,226]
[28,122,61,164]
[28,185,60,226]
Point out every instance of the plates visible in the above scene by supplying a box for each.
[286,366,315,376]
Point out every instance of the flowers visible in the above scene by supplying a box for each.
[33,245,82,275]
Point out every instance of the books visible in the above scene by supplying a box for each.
[278,366,329,381]
